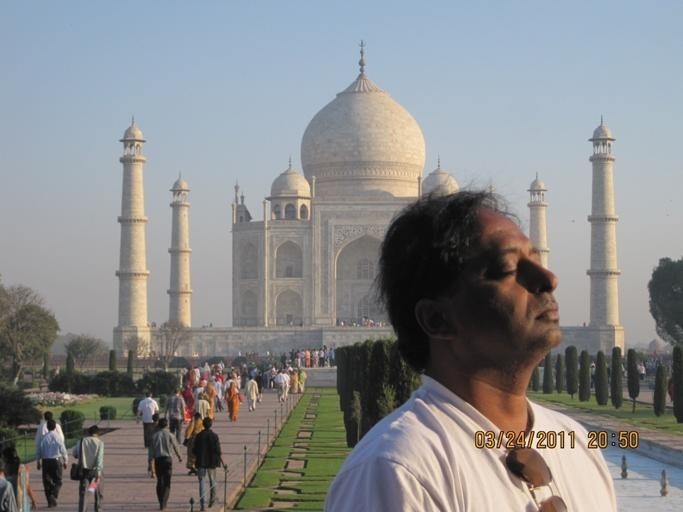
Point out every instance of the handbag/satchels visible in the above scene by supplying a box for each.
[183,439,192,447]
[71,462,83,479]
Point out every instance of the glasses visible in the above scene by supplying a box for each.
[506,444,568,512]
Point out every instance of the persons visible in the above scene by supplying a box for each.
[36,420,68,507]
[146,412,161,441]
[1,443,37,512]
[148,417,183,511]
[589,359,595,373]
[320,185,623,510]
[39,410,66,447]
[135,390,159,449]
[0,467,18,511]
[287,319,294,326]
[191,415,229,511]
[280,343,335,368]
[270,363,307,402]
[337,315,392,326]
[183,412,204,475]
[163,360,264,445]
[70,425,105,512]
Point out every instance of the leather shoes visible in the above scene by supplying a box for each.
[47,495,57,507]
